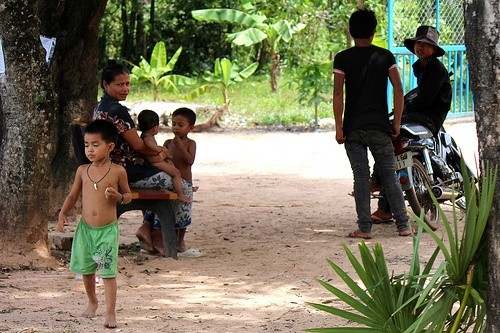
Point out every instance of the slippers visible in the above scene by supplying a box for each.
[348,189,383,198]
[177,247,206,257]
[348,229,371,239]
[398,226,417,236]
[357,211,394,223]
[140,246,161,255]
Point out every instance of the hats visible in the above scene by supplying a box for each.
[404,24,445,57]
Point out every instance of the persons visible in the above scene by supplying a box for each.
[138,110,199,202]
[93,64,194,255]
[347,26,452,224]
[333,9,419,239]
[163,107,196,252]
[59,119,132,327]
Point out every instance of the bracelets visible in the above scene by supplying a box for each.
[160,153,164,162]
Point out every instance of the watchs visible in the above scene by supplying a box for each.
[116,194,124,205]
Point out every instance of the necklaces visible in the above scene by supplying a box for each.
[87,160,112,190]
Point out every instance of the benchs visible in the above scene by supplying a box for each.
[71,123,176,259]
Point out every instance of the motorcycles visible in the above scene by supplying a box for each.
[388,70,479,231]
